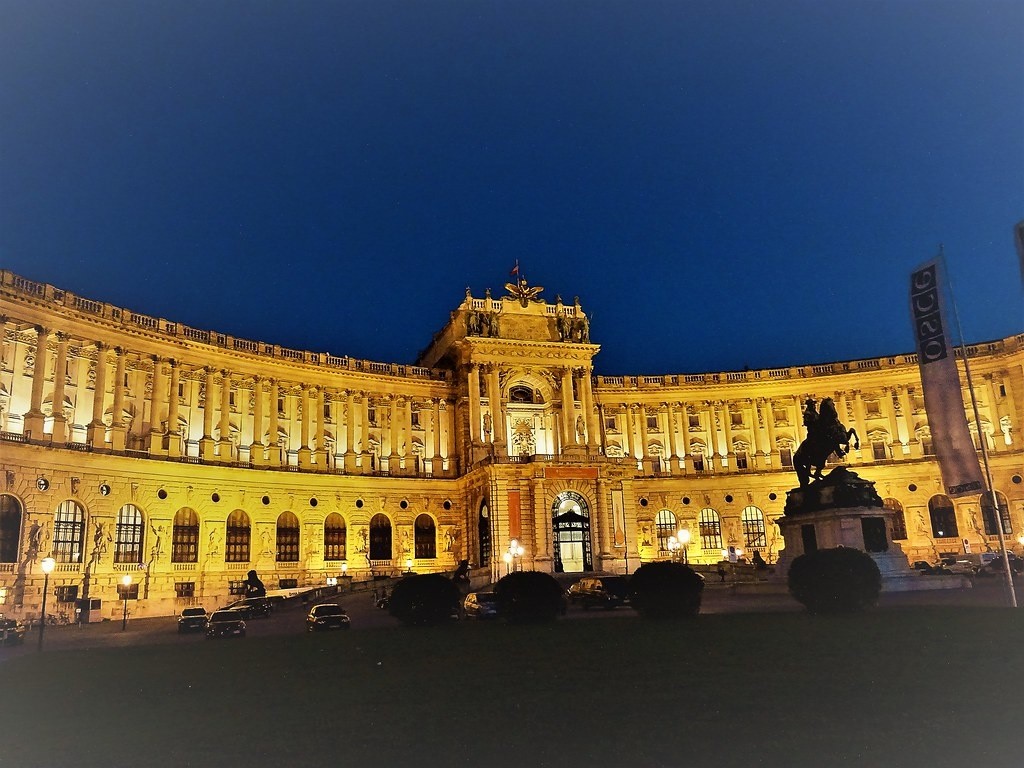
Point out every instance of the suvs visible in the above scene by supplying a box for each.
[562,575,632,611]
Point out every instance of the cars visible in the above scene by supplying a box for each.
[307,603,350,633]
[219,597,274,619]
[204,609,247,640]
[0,618,25,645]
[462,591,497,619]
[910,550,1024,575]
[178,607,209,633]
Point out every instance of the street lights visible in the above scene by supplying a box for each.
[120,574,132,631]
[37,557,57,650]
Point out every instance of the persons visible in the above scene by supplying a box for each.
[962,538,992,553]
[357,531,365,552]
[728,547,737,560]
[803,399,831,446]
[153,525,167,553]
[966,507,977,528]
[576,414,586,435]
[400,531,410,553]
[209,528,221,553]
[483,410,493,432]
[914,511,925,532]
[445,531,452,551]
[671,548,684,564]
[471,308,501,336]
[560,314,588,342]
[35,523,48,552]
[260,527,272,552]
[97,522,112,555]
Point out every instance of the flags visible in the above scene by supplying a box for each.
[510,266,518,276]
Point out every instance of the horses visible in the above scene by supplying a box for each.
[793,397,859,489]
